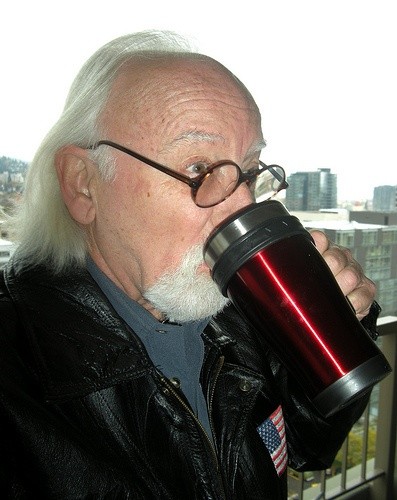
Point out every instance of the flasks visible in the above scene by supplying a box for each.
[202,201,392,417]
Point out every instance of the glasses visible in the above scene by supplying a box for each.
[81,138,289,209]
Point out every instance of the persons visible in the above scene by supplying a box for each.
[0,30,381,500]
